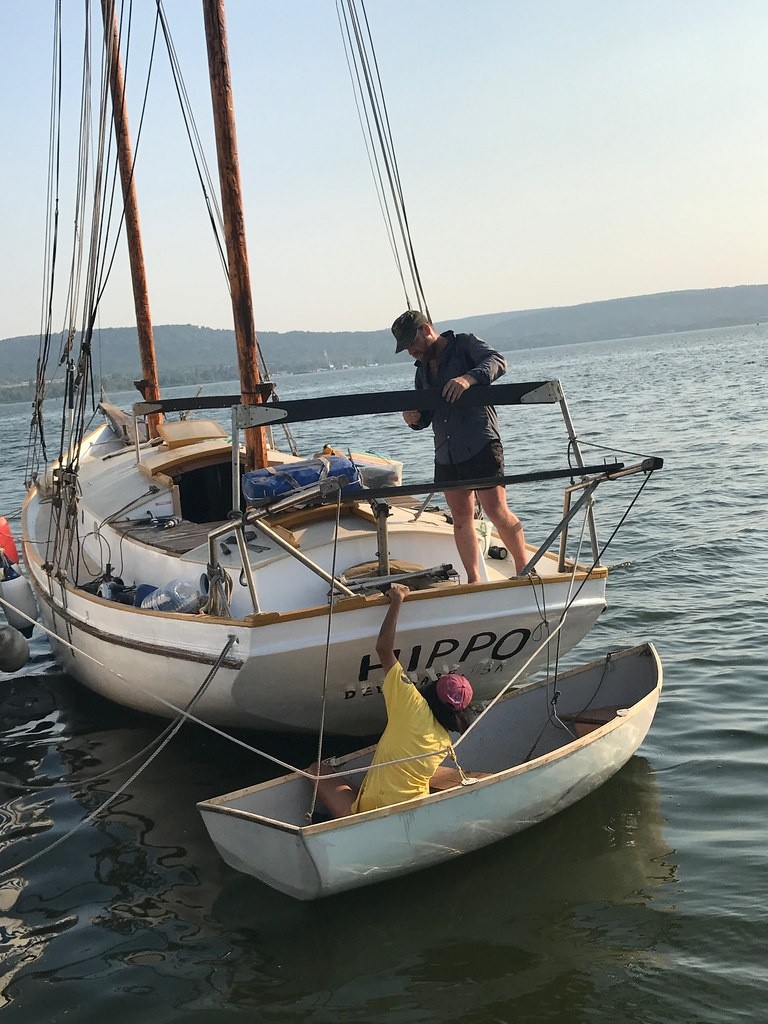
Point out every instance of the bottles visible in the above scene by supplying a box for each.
[141,578,199,612]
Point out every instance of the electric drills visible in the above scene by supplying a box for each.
[150,515,181,528]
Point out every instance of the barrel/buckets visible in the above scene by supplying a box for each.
[473,519,494,560]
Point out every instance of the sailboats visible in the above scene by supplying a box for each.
[0,2,685,744]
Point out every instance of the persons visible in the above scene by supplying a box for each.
[391,310,528,584]
[307,586,474,820]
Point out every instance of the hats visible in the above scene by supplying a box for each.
[392,310,428,354]
[436,674,474,710]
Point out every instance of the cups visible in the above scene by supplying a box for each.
[488,547,507,559]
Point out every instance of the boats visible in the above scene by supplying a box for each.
[195,635,666,901]
